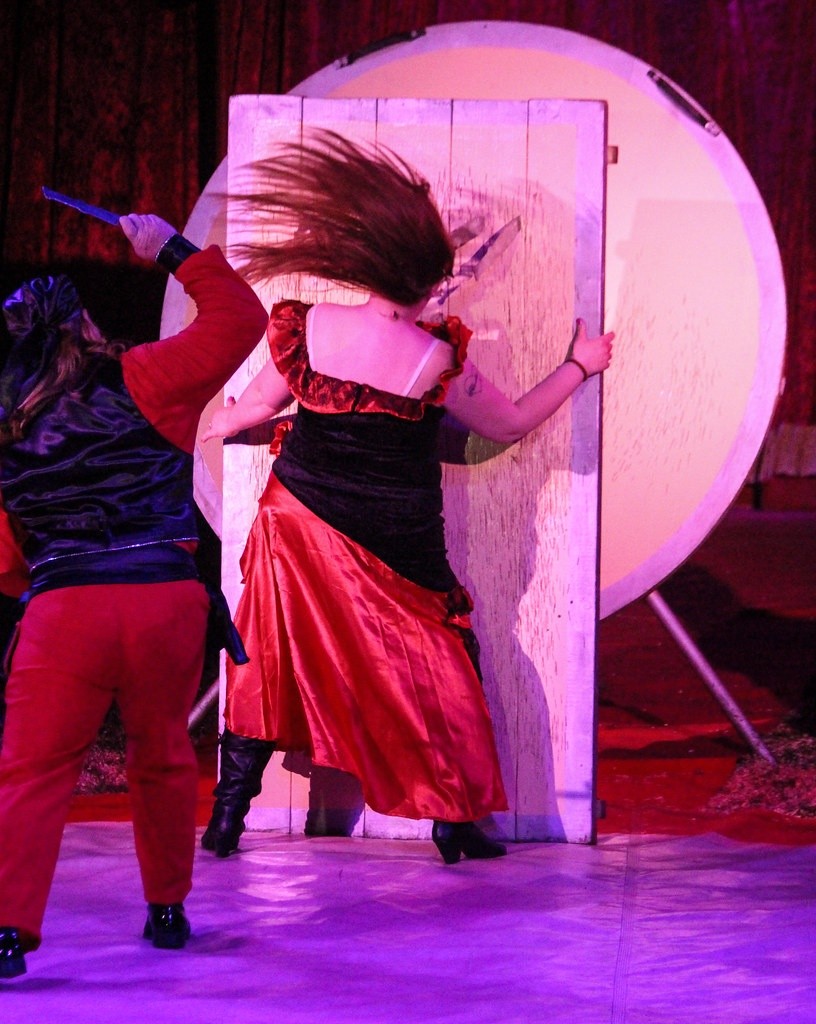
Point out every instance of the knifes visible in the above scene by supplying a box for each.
[440,216,522,305]
[41,184,127,227]
[446,216,486,250]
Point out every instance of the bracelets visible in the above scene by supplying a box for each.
[563,358,587,382]
[155,234,175,263]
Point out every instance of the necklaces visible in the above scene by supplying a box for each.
[378,310,403,322]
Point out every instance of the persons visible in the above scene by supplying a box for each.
[0,211,270,978]
[201,127,616,866]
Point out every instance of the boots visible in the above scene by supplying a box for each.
[201,726,278,859]
[432,812,507,864]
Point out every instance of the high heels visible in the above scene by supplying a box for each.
[144,903,189,950]
[0,928,26,979]
